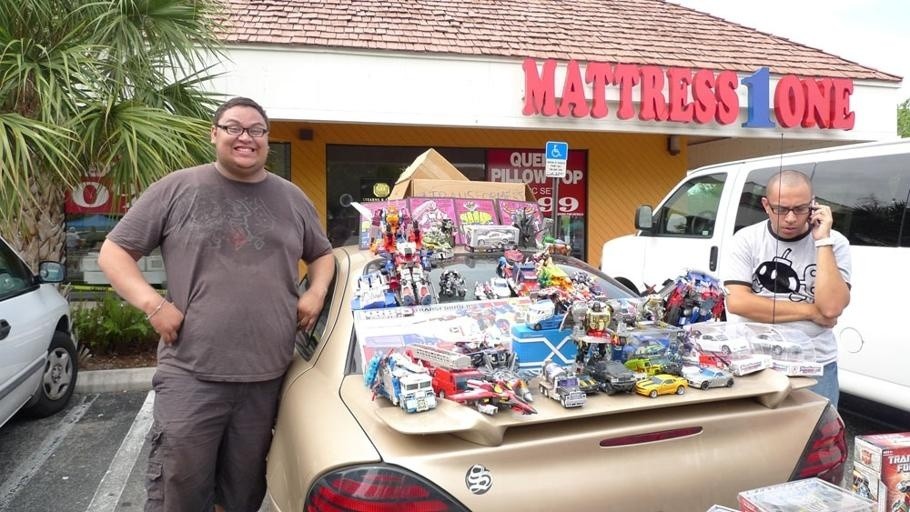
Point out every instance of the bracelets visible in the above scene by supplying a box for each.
[142,297,167,320]
[814,237,836,247]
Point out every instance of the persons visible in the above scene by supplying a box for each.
[94,95,338,510]
[721,169,854,416]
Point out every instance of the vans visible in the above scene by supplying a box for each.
[601,135,910,437]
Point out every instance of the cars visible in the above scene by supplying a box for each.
[0,232,80,436]
[258,241,853,512]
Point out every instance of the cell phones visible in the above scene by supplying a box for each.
[812,195,821,226]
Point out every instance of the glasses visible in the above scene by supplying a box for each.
[215,124,268,137]
[761,195,812,215]
